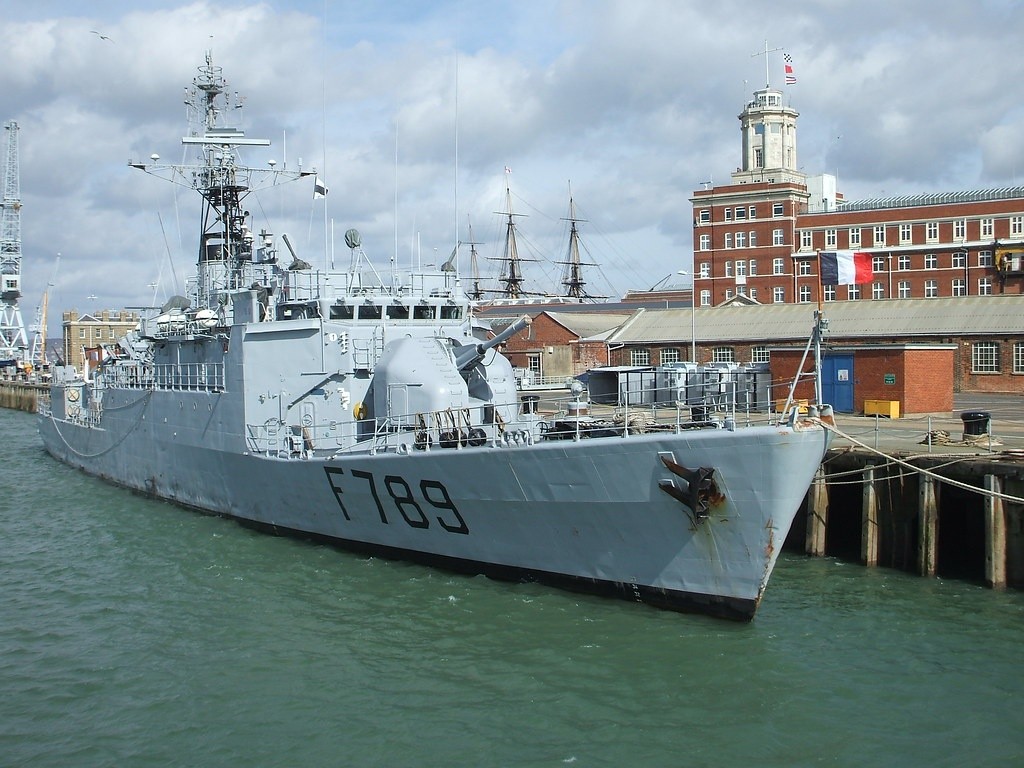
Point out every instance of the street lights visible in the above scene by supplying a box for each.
[678,271,695,363]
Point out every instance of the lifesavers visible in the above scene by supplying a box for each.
[414,428,487,451]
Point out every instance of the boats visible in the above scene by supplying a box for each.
[37,37,834,623]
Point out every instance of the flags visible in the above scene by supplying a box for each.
[314,178,330,199]
[784,51,796,86]
[505,167,512,174]
[820,251,875,285]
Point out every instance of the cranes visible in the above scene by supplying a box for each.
[0,120,24,299]
[28,252,60,333]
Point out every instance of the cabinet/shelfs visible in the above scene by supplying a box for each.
[776,399,808,414]
[864,400,899,419]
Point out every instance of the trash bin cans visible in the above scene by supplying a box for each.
[960,412,991,445]
[567,402,588,416]
[689,403,712,427]
[520,396,540,414]
